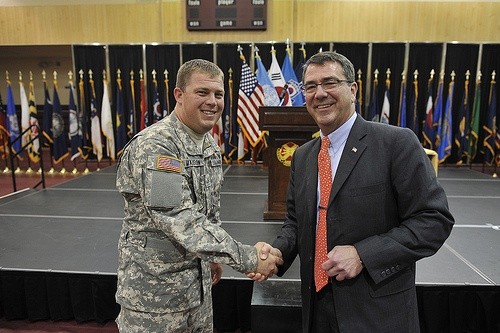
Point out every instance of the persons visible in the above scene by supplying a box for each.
[114,59,283,333]
[246,51,455,333]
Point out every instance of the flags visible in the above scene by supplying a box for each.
[210,52,308,165]
[41,79,176,163]
[0,80,41,164]
[355,80,499,168]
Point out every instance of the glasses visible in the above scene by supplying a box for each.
[300,79,354,95]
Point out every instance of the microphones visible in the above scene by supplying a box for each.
[279,81,290,98]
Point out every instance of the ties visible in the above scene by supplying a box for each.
[313,136,332,293]
